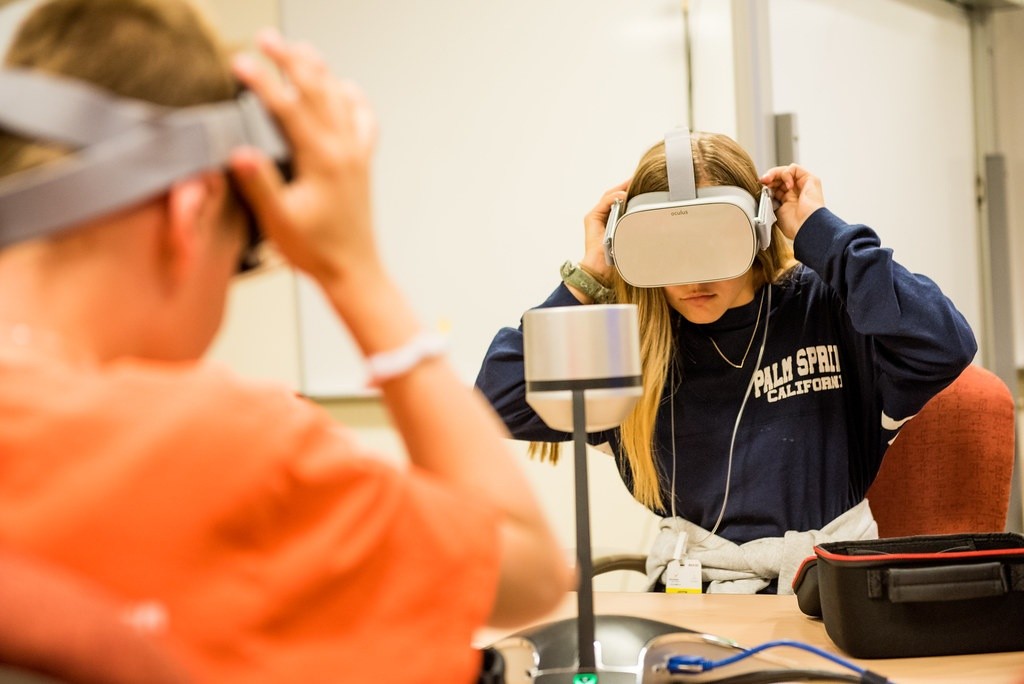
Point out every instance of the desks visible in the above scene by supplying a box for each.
[477,588,1024,684]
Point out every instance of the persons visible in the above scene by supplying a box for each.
[475,133,978,594]
[0,0,572,684]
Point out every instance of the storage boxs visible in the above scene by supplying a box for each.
[792,528,1024,659]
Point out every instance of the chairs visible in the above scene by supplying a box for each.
[861,362,1014,544]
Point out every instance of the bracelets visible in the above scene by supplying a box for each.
[362,331,447,384]
[560,261,615,304]
